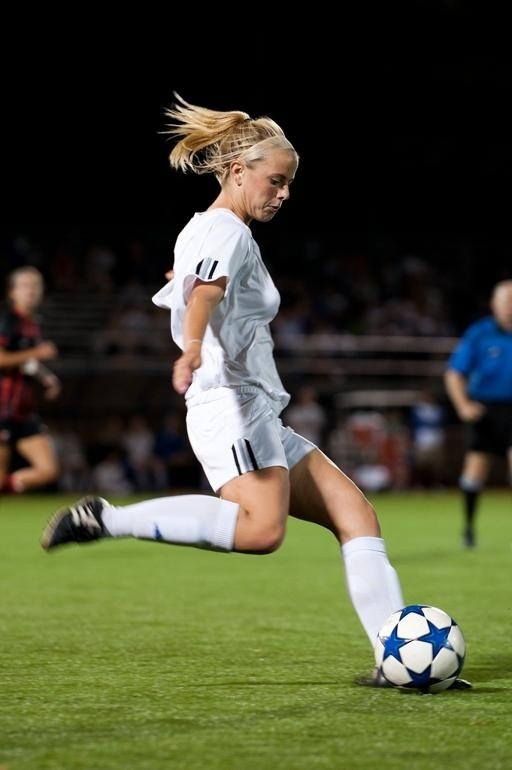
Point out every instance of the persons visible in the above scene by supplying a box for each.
[40,89,470,698]
[0,230,512,548]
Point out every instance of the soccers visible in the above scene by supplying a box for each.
[374,605,465,692]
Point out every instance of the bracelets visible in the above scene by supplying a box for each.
[183,339,202,348]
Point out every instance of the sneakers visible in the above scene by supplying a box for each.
[372,672,471,687]
[39,495,106,551]
